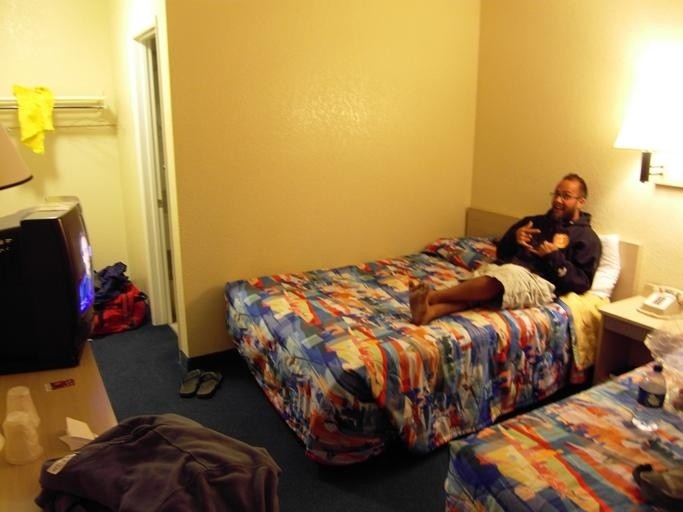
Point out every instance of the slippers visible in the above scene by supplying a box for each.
[180,370,203,396]
[197,371,222,397]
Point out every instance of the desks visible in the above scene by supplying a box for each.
[0,340,119,512]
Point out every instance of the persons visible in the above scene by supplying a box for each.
[409,174,602,326]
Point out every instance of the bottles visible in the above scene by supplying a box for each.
[632,358,666,431]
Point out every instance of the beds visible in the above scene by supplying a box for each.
[223,206,645,479]
[445,360,683,512]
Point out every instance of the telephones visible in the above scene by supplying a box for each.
[636,283,683,319]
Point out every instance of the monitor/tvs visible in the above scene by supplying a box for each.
[0,197,95,376]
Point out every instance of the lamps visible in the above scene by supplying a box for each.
[614,39,683,190]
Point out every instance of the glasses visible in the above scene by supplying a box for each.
[550,192,580,201]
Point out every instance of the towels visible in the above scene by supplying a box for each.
[13,83,55,153]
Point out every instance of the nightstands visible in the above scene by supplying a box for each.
[593,295,683,384]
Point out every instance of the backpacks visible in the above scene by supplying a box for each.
[94,262,130,305]
